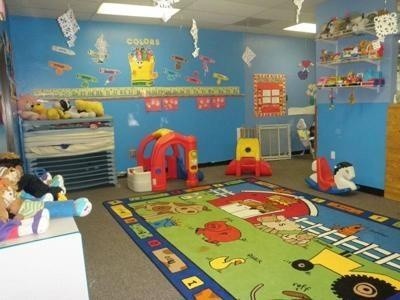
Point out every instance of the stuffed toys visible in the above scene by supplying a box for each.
[17,94,108,131]
[0,165,93,241]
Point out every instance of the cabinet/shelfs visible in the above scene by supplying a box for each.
[18,115,116,193]
[314,24,381,95]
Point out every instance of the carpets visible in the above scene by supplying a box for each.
[102,176,400,300]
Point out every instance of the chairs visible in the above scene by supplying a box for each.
[235,137,261,176]
[296,119,315,158]
